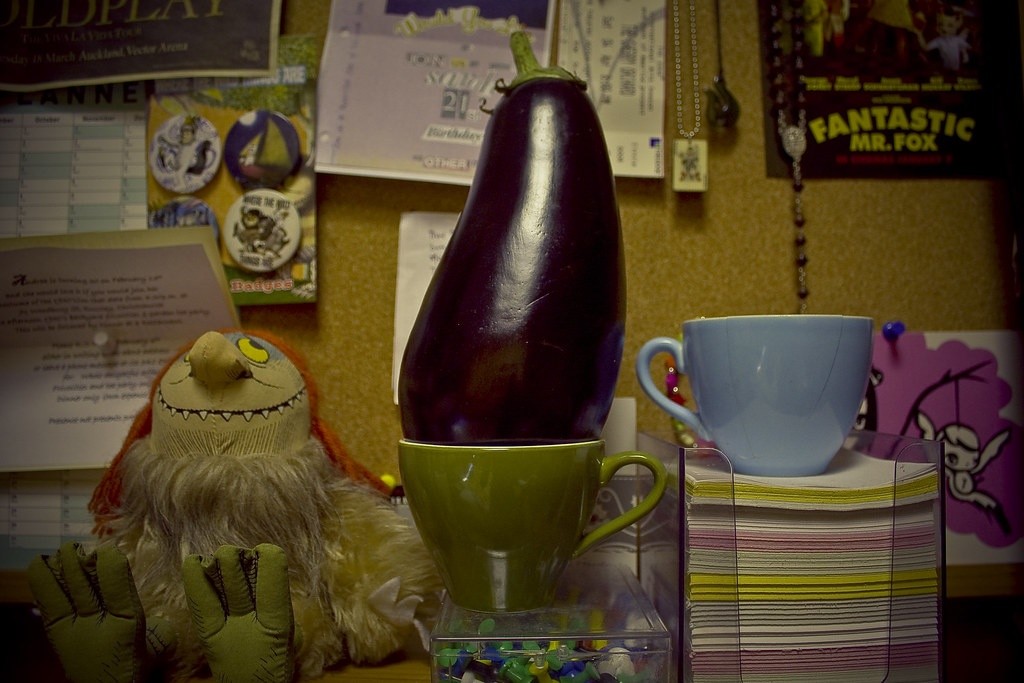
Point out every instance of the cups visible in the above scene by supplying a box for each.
[636,311,874,479]
[396,428,669,615]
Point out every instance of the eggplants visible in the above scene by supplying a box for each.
[398,77,626,445]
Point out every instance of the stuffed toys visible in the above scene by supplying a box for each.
[0,329,439,683]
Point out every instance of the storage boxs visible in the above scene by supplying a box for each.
[426,559,671,683]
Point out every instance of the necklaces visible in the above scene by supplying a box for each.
[703,0,740,129]
[670,0,707,191]
[771,0,811,314]
